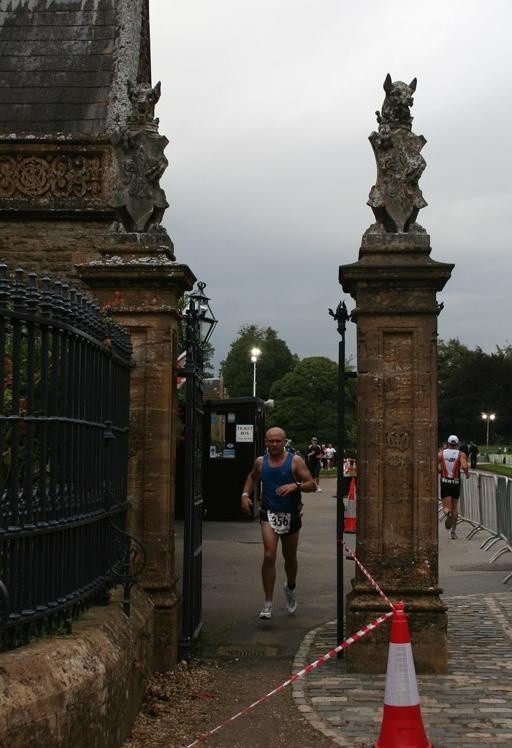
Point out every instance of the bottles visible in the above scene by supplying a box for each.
[447,435,459,446]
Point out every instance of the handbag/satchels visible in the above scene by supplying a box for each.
[242,492,250,497]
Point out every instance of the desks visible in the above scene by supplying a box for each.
[482,411,495,453]
[250,347,261,397]
[180,281,218,349]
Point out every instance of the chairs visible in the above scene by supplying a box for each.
[450,532,457,539]
[444,510,453,530]
[283,581,297,615]
[259,601,273,620]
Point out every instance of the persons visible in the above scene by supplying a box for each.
[438,434,470,540]
[439,441,479,468]
[241,427,317,620]
[285,437,337,493]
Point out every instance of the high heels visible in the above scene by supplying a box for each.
[344,482,358,534]
[376,604,434,748]
